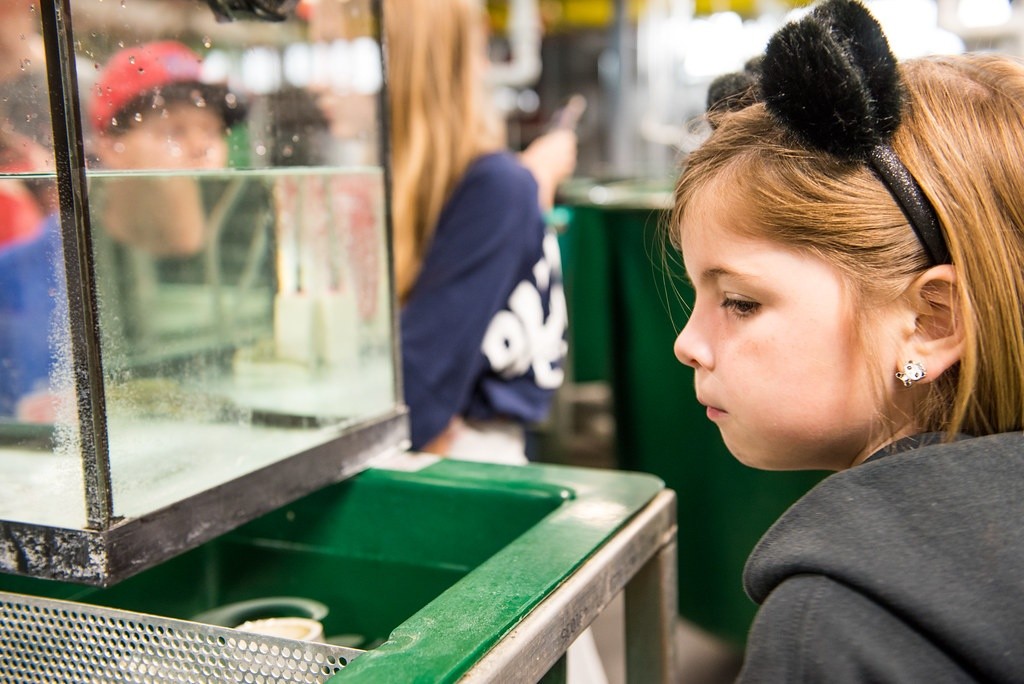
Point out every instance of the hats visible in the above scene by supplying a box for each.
[87,42,249,135]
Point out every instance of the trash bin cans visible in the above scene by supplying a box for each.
[556,173,840,649]
[1,452,666,684]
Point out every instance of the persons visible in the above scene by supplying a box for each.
[664,0,1024,684]
[0,37,231,428]
[306,0,577,466]
